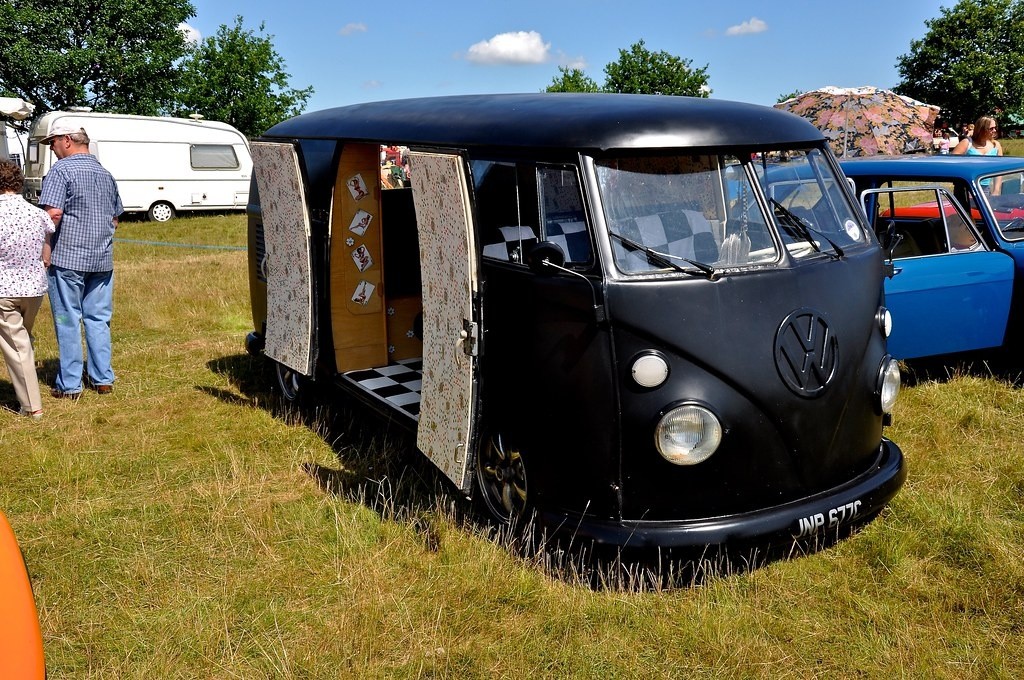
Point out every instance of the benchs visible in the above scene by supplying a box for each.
[476,205,720,272]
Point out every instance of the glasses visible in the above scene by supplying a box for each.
[49,136,65,146]
[988,126,997,131]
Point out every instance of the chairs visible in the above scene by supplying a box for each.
[879,227,920,258]
[923,218,945,253]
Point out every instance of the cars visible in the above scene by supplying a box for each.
[723,148,1024,384]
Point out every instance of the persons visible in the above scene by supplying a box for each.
[952,117,1004,198]
[35,127,124,399]
[938,124,975,155]
[0,157,53,419]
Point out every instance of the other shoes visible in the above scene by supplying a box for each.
[85,375,112,393]
[51,387,80,400]
[6,400,42,419]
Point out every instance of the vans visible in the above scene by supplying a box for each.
[22,105,255,222]
[241,88,909,592]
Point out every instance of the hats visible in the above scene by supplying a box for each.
[37,121,88,145]
